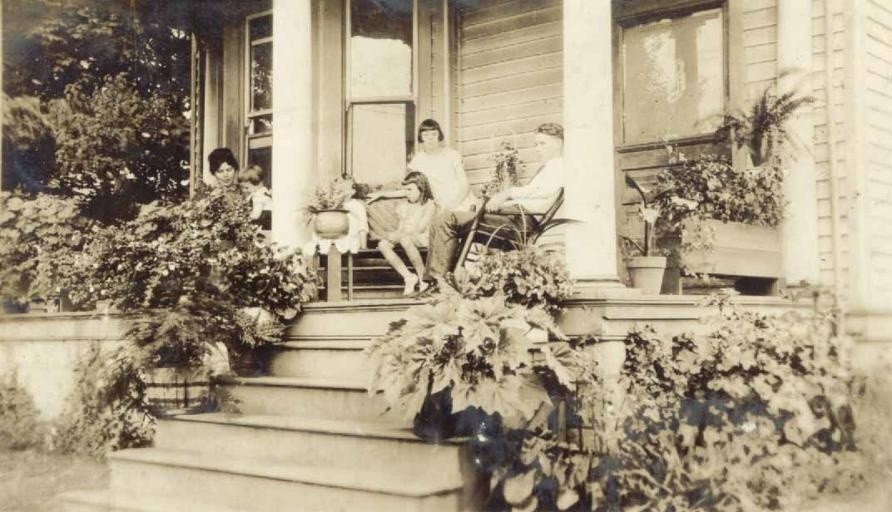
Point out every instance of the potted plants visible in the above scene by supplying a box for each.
[297,174,358,240]
[460,243,583,344]
[355,270,583,441]
[211,228,325,347]
[649,137,794,288]
[616,170,699,295]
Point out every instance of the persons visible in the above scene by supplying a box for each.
[422,123,566,284]
[197,147,271,232]
[406,119,479,214]
[365,171,439,296]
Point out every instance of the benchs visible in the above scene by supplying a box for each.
[315,197,430,301]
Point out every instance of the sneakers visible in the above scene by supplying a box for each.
[403,273,429,297]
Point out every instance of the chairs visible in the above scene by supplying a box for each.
[454,186,564,270]
